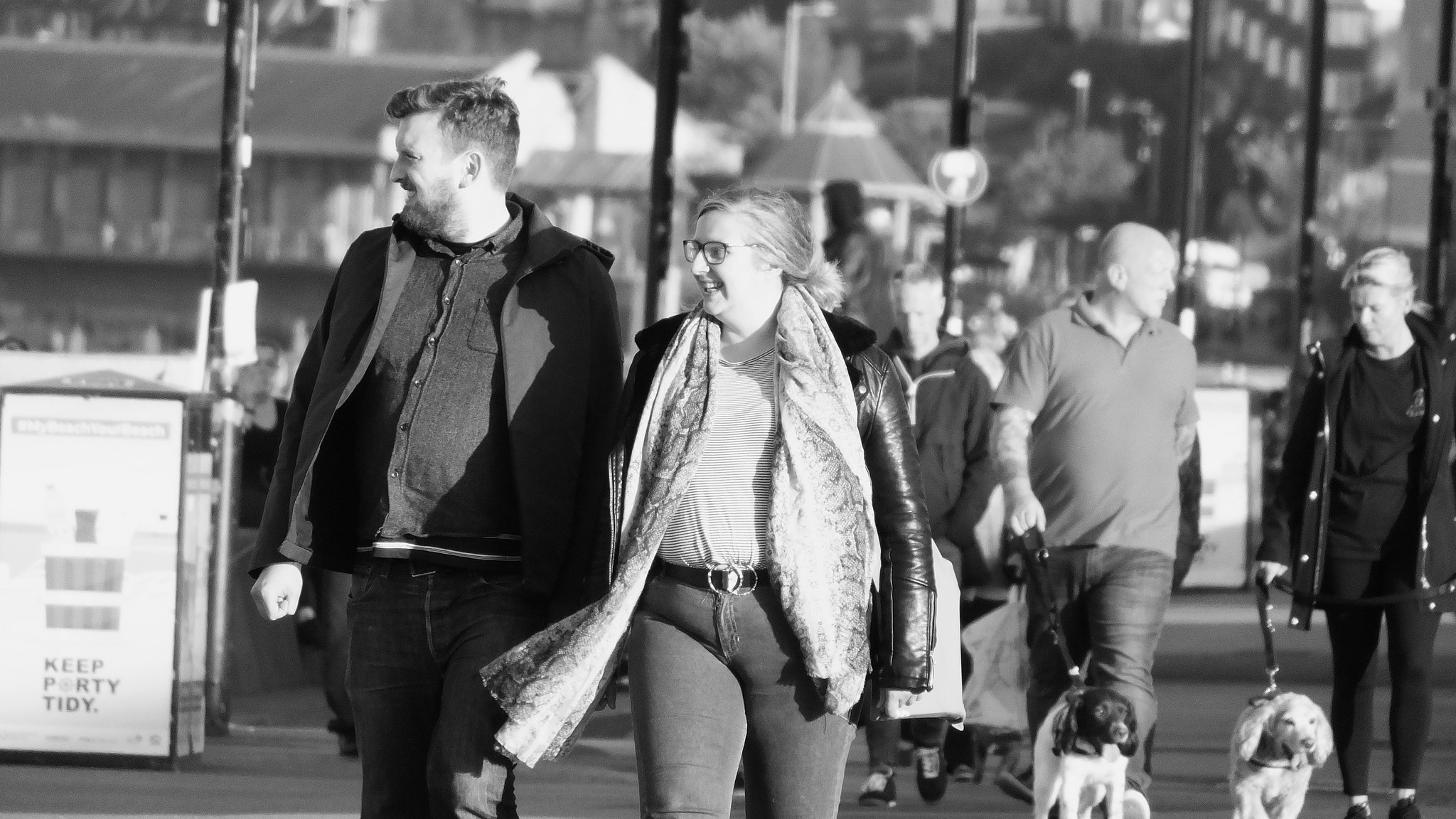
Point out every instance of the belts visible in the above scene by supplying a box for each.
[355,551,459,578]
[651,555,769,596]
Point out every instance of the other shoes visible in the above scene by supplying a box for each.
[1342,801,1372,819]
[1121,787,1150,819]
[858,764,897,806]
[954,762,975,782]
[1000,764,1036,804]
[1388,795,1422,819]
[913,746,947,805]
[338,720,358,756]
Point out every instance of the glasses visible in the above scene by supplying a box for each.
[683,239,768,264]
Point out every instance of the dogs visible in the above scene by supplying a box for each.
[1229,692,1333,819]
[1030,684,1151,819]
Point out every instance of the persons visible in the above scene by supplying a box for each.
[851,262,1000,808]
[250,75,625,819]
[590,188,936,819]
[985,221,1204,819]
[1256,248,1456,819]
